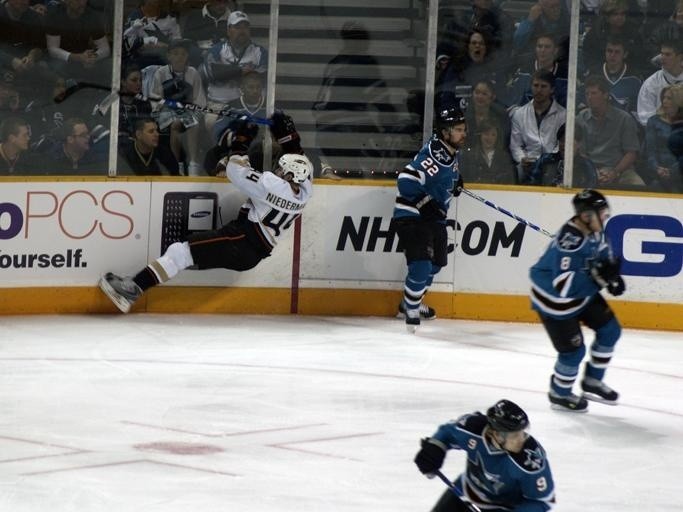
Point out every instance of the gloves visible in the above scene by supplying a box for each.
[268,111,301,150]
[417,193,443,220]
[594,258,626,297]
[227,114,260,152]
[415,438,446,473]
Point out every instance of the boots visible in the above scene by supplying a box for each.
[548,390,587,410]
[105,273,143,303]
[582,379,618,400]
[396,302,435,325]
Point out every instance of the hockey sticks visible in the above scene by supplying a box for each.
[55,81,274,127]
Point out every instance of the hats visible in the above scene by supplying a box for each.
[227,11,249,25]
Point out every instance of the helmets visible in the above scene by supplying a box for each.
[278,153,311,184]
[438,106,465,123]
[572,189,609,211]
[488,400,528,431]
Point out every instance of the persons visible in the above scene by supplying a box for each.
[414,399,556,512]
[393,107,467,324]
[412,2,681,191]
[98,108,314,314]
[529,190,626,412]
[1,1,267,176]
[310,20,399,184]
